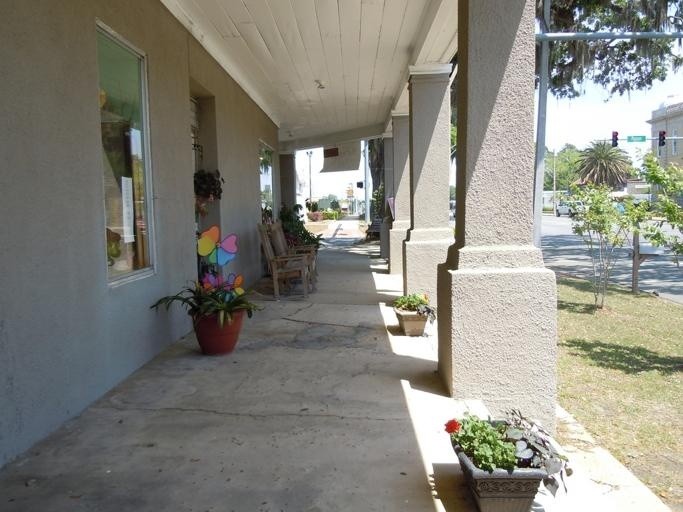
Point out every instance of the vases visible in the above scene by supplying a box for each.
[395,310,430,337]
[448,433,550,512]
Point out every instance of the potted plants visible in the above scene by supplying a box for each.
[150,278,268,356]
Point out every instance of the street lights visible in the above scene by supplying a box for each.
[306,152,312,202]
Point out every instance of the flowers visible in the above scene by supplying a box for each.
[393,291,437,325]
[444,407,573,498]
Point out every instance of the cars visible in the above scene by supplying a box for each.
[556,201,585,217]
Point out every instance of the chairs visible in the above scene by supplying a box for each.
[258,222,318,299]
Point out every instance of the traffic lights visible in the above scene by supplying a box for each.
[612,132,618,147]
[659,131,666,146]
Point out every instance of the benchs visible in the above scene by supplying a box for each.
[365,219,383,241]
